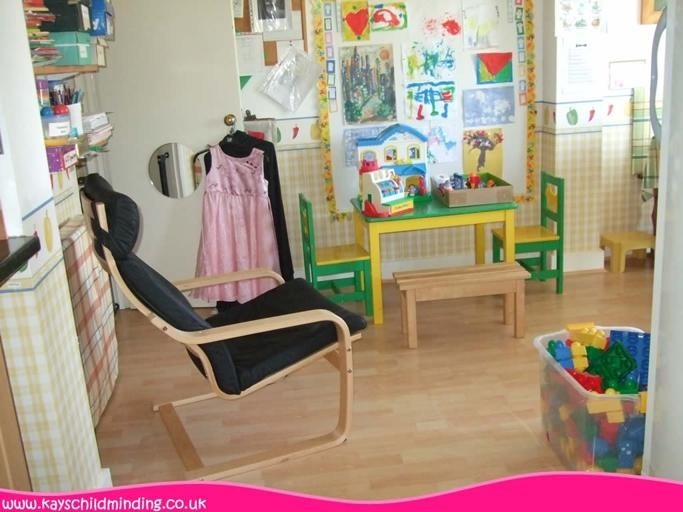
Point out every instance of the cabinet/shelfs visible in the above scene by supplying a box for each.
[35,65,108,150]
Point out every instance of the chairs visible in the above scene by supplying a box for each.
[78,172,370,481]
[493,172,566,294]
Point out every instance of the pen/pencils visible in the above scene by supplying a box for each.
[50,83,83,104]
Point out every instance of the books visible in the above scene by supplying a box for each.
[25,0,60,68]
[83,111,114,147]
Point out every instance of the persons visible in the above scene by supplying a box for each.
[264,0,285,19]
[535,319,651,474]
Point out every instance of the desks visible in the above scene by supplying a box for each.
[348,194,517,325]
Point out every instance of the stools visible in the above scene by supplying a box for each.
[599,228,655,274]
[391,260,532,348]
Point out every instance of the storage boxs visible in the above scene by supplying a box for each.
[531,326,647,475]
